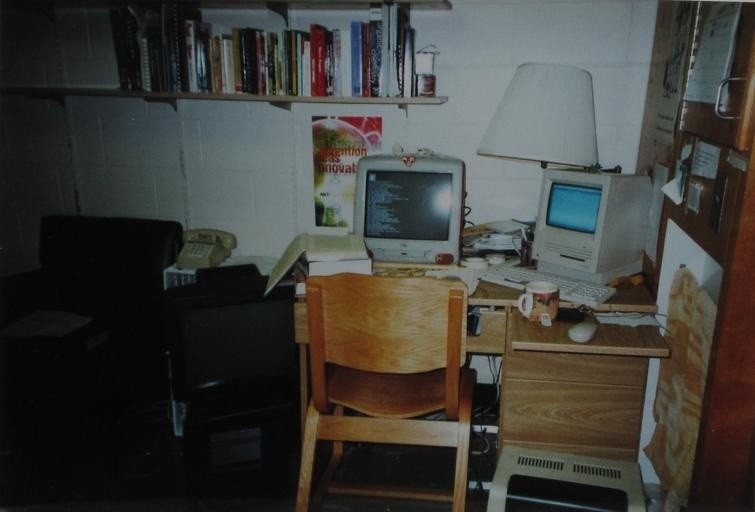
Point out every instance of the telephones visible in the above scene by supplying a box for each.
[176,229,237,270]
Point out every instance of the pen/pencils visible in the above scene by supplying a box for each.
[521,226,533,266]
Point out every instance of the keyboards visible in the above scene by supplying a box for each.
[481,263,617,307]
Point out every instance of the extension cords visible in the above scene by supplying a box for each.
[472,424,499,434]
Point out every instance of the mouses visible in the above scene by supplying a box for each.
[569,321,599,343]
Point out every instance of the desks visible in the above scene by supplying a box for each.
[294,225,671,461]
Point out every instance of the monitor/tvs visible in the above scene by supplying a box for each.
[353,155,465,265]
[530,168,651,285]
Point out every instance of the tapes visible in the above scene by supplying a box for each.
[461,254,506,271]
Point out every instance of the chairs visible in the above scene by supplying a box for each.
[295,272,477,512]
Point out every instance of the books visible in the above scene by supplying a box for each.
[297,254,374,277]
[461,217,531,251]
[261,232,369,296]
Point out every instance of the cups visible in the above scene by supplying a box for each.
[517,280,561,323]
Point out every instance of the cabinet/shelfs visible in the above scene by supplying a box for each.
[0,0,452,110]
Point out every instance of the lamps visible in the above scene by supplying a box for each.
[476,62,599,171]
